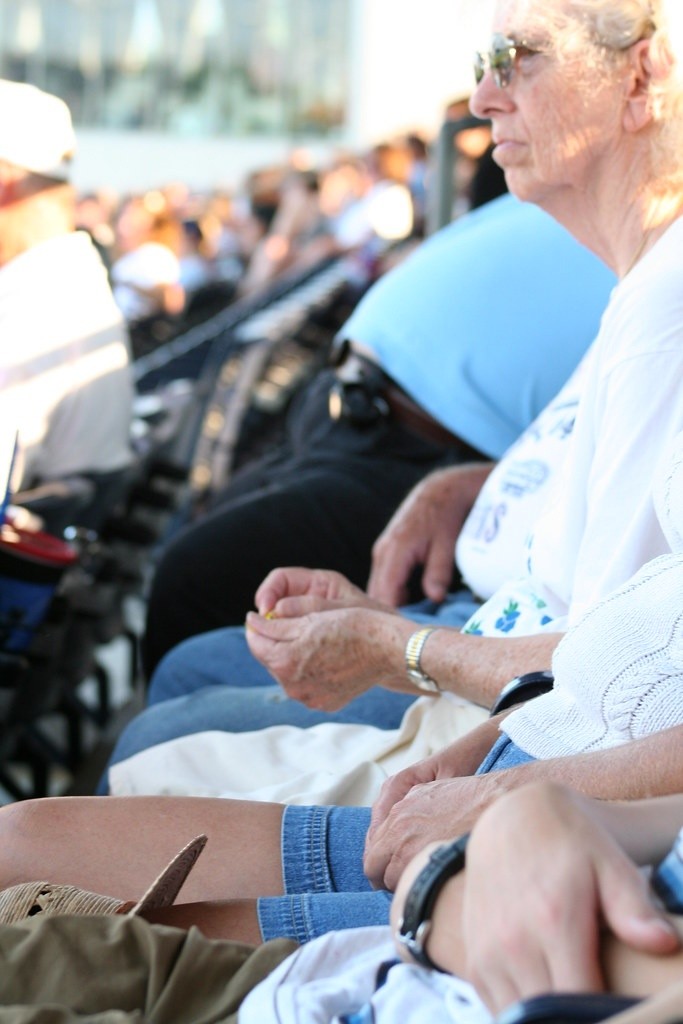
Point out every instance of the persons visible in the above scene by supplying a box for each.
[0,0,683,1024]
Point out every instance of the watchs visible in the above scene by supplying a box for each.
[405,626,440,693]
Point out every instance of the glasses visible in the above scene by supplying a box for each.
[472,30,624,89]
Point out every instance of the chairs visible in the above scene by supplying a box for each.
[0,416,200,803]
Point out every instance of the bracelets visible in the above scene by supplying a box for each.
[397,833,468,974]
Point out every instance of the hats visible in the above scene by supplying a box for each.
[0,834,208,927]
[0,78,74,181]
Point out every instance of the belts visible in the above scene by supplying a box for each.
[341,354,490,463]
